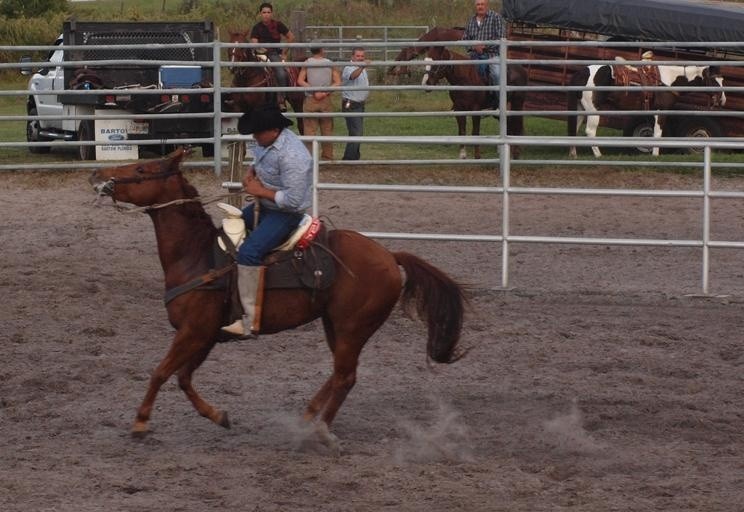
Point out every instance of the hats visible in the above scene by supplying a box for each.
[237,101,293,135]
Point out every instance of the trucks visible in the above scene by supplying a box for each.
[501,0,744,157]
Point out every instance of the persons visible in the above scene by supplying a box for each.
[250,2,295,113]
[295,46,342,167]
[220,103,313,340]
[462,0,507,120]
[341,47,371,161]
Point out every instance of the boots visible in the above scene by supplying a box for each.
[221,264,266,340]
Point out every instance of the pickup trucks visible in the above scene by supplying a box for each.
[19,20,246,160]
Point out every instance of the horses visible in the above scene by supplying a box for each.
[386,26,469,76]
[420,46,529,160]
[566,64,728,160]
[227,27,308,136]
[89,143,474,443]
